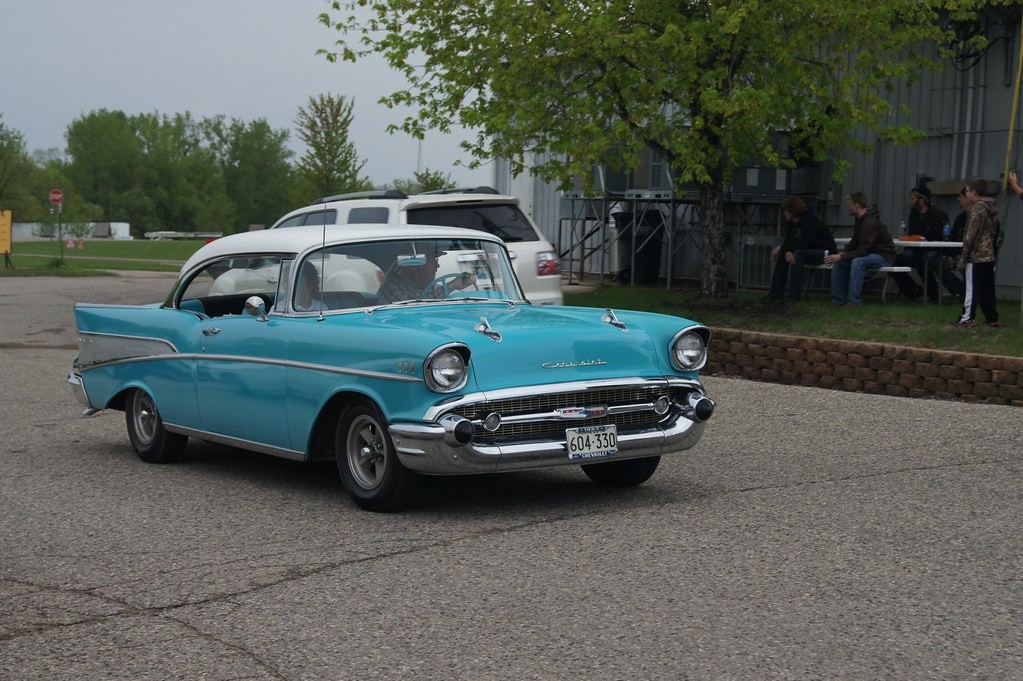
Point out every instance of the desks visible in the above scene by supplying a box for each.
[833,239,965,307]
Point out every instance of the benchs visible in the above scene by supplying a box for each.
[803,262,912,304]
[181,290,365,317]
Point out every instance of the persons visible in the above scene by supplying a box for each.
[937,186,1003,299]
[823,192,899,306]
[1009,170,1023,200]
[758,194,840,304]
[265,260,330,314]
[951,178,1001,328]
[377,242,476,307]
[891,185,952,305]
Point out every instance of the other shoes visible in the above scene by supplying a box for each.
[765,291,784,300]
[822,299,834,305]
[843,303,854,308]
[782,293,799,301]
[983,321,999,327]
[952,320,977,328]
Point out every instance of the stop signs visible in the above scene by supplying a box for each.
[49,190,63,203]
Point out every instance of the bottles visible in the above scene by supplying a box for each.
[899,221,907,238]
[943,221,951,242]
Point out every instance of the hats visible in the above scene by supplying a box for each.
[430,248,447,258]
[910,186,932,205]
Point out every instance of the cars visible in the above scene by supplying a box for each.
[67,223,717,512]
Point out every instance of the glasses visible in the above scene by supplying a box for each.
[963,189,972,194]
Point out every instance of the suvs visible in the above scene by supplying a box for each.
[208,186,563,309]
